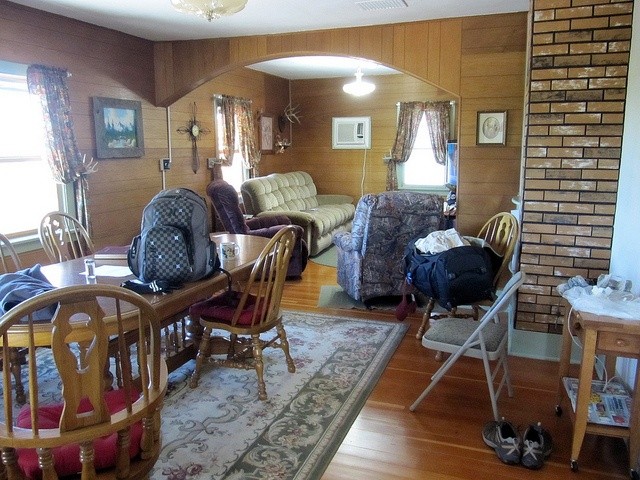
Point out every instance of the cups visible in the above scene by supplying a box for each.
[220,242,240,261]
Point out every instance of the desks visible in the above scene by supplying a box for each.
[556,294,639,478]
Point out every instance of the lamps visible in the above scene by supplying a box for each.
[343,65,376,97]
[168,0,246,23]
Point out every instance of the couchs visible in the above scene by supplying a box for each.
[331,194,443,306]
[241,170,355,257]
[206,179,308,277]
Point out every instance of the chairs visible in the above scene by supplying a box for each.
[37,211,96,264]
[190,226,303,401]
[416,211,518,363]
[0,284,169,480]
[408,271,524,424]
[0,233,24,274]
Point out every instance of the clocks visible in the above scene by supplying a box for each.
[191,123,201,137]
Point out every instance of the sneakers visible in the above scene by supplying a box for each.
[523,424,552,469]
[482,420,522,466]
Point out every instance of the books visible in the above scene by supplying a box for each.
[561,375,632,428]
[94,246,130,260]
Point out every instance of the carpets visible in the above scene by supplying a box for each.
[317,285,400,310]
[2,307,409,480]
[310,242,339,267]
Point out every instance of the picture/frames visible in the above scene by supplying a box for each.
[258,113,277,155]
[92,96,146,160]
[476,110,508,147]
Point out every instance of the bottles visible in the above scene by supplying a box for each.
[84,259,96,277]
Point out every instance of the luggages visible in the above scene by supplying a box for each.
[404,235,505,313]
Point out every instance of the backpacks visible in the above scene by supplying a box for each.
[127,188,232,292]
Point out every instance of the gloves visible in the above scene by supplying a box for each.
[119,279,170,295]
